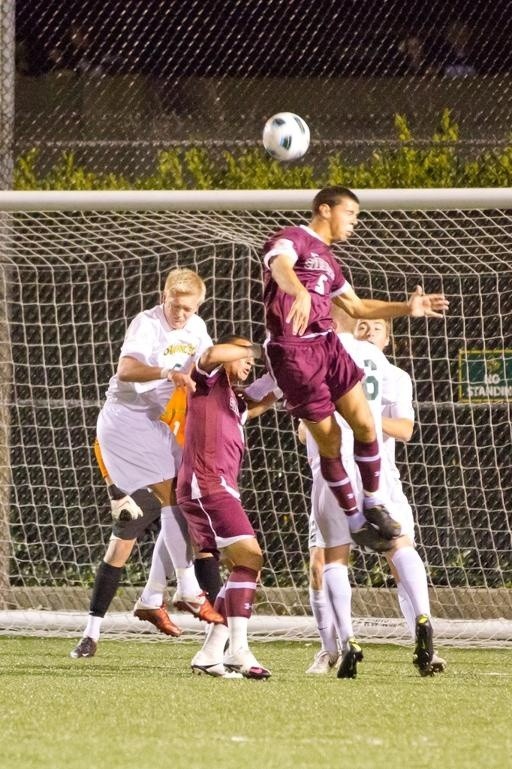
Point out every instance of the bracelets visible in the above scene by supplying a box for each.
[273,385,283,399]
[252,341,262,360]
[160,366,169,380]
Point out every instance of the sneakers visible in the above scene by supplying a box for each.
[72,639,99,659]
[192,651,244,680]
[170,588,224,626]
[413,617,445,678]
[362,501,401,539]
[340,638,362,678]
[224,651,273,680]
[133,598,182,637]
[349,522,396,551]
[308,649,341,675]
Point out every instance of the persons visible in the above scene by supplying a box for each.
[70,386,188,658]
[260,187,449,552]
[245,302,433,678]
[96,269,226,635]
[299,317,447,675]
[175,335,284,679]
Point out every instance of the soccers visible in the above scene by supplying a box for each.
[263,112,310,159]
